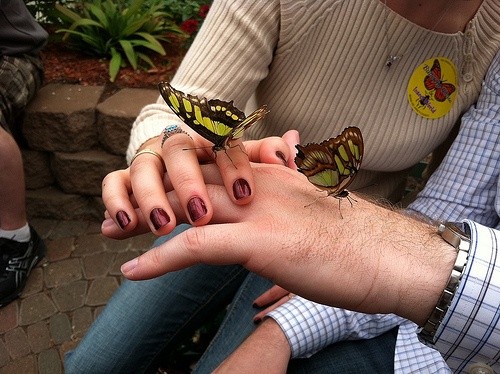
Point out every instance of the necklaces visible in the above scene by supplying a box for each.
[384,0,451,68]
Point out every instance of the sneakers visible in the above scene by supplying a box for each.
[0,225,46,308]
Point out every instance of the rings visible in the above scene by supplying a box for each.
[161,124,194,151]
[130,149,166,176]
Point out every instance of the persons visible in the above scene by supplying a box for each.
[64,0,500,374]
[0,0,49,310]
[100,160,500,374]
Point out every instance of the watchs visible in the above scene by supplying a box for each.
[415,221,471,342]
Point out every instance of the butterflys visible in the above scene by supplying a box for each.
[294,126,364,219]
[159,81,271,170]
